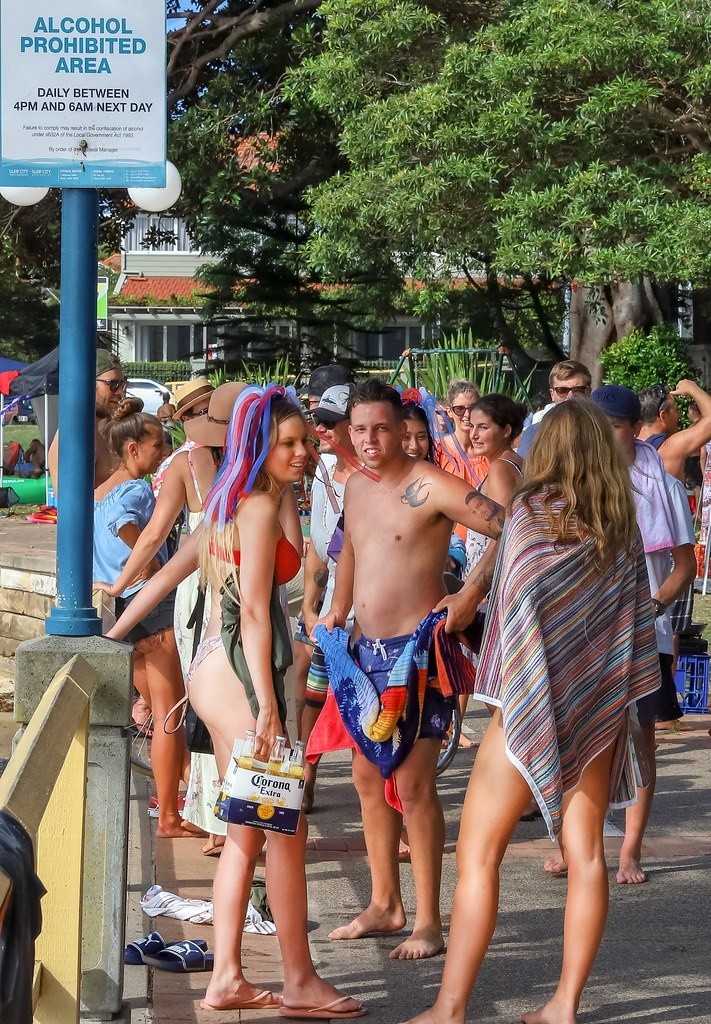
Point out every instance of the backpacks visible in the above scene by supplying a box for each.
[3,441,25,475]
[23,439,45,478]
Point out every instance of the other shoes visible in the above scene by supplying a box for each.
[149,792,190,818]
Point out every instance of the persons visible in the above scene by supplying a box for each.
[636,380,711,730]
[309,379,507,959]
[327,365,549,860]
[404,400,663,1024]
[15,440,44,478]
[517,361,592,459]
[543,385,697,884]
[107,384,366,1018]
[47,348,410,860]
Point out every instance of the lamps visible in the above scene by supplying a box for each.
[0,487,20,518]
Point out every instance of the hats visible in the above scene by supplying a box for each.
[304,384,359,421]
[171,377,216,420]
[294,364,354,397]
[95,349,121,377]
[588,385,641,421]
[183,382,250,448]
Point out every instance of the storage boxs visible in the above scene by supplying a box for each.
[215,738,307,835]
[672,650,711,715]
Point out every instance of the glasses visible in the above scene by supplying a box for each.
[655,383,667,417]
[313,415,349,430]
[98,380,130,392]
[303,398,322,409]
[554,386,588,398]
[449,404,474,418]
[187,408,209,417]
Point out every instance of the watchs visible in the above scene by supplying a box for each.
[652,598,666,618]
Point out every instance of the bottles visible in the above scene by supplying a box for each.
[237,729,257,771]
[286,739,306,780]
[264,735,286,777]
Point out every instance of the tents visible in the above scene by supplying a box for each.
[0,348,59,506]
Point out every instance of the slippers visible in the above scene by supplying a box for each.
[199,980,282,1011]
[127,719,153,739]
[123,933,179,964]
[141,941,218,973]
[277,981,368,1018]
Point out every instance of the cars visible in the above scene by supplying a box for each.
[124,378,175,418]
